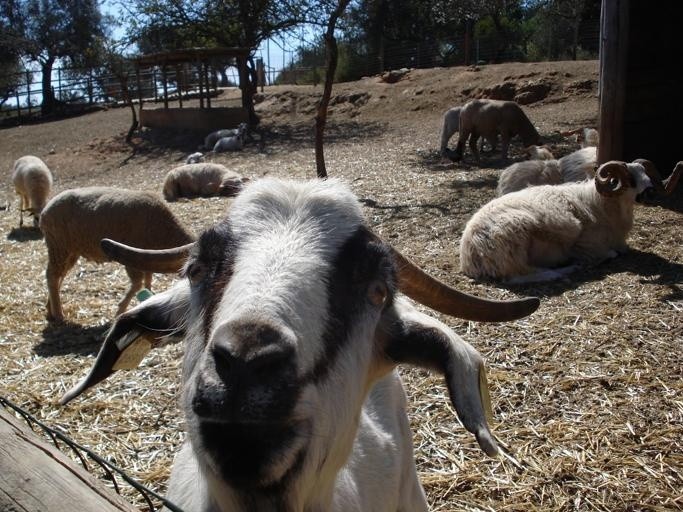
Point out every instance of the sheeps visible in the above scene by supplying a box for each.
[58,173,541,511]
[11,154,54,228]
[440,98,542,164]
[37,185,197,324]
[163,121,248,202]
[459,158,683,286]
[497,127,600,197]
